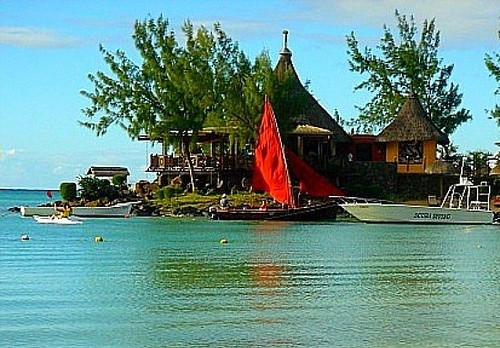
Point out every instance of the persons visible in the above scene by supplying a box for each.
[220,193,230,210]
[259,199,268,212]
[50,202,73,220]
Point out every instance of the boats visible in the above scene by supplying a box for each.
[20,200,141,217]
[328,181,495,224]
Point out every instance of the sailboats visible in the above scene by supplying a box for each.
[206,95,338,221]
